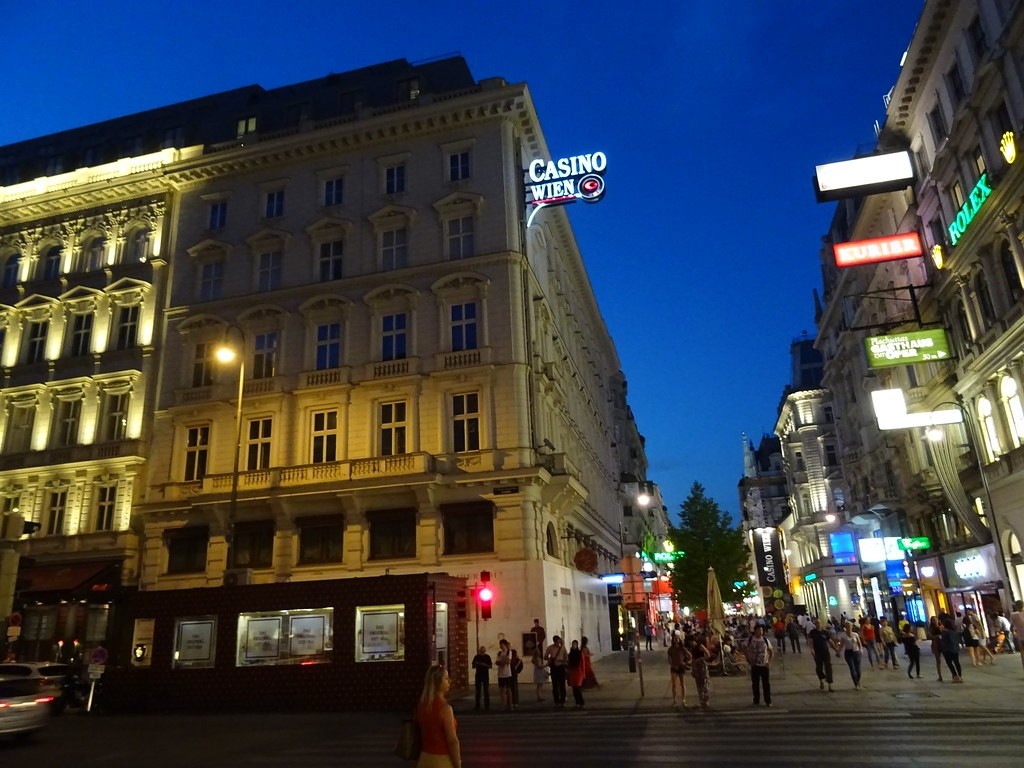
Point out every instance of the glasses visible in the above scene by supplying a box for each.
[438,664,443,670]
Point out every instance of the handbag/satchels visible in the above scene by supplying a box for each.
[391,703,421,760]
[549,661,555,668]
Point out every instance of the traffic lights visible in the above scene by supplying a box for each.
[481,588,492,619]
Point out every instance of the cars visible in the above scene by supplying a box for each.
[0,660,71,742]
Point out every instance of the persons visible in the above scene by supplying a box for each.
[567,640,586,711]
[644,599,1024,712]
[472,646,493,713]
[580,635,603,691]
[413,665,462,768]
[495,639,522,712]
[532,635,569,708]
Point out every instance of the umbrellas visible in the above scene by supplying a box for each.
[707,566,727,677]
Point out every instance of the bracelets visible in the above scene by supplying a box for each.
[454,760,462,767]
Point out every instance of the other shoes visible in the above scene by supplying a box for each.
[767,703,773,707]
[952,678,962,683]
[499,704,520,712]
[820,683,823,689]
[673,704,676,708]
[485,707,490,711]
[472,707,480,710]
[753,704,759,707]
[682,702,687,707]
[536,698,545,701]
[937,678,942,681]
[909,674,913,679]
[829,690,834,692]
[916,675,924,678]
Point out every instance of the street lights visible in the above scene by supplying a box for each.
[210,321,249,569]
[922,400,1017,603]
[653,533,674,648]
[617,473,653,696]
[824,498,872,612]
